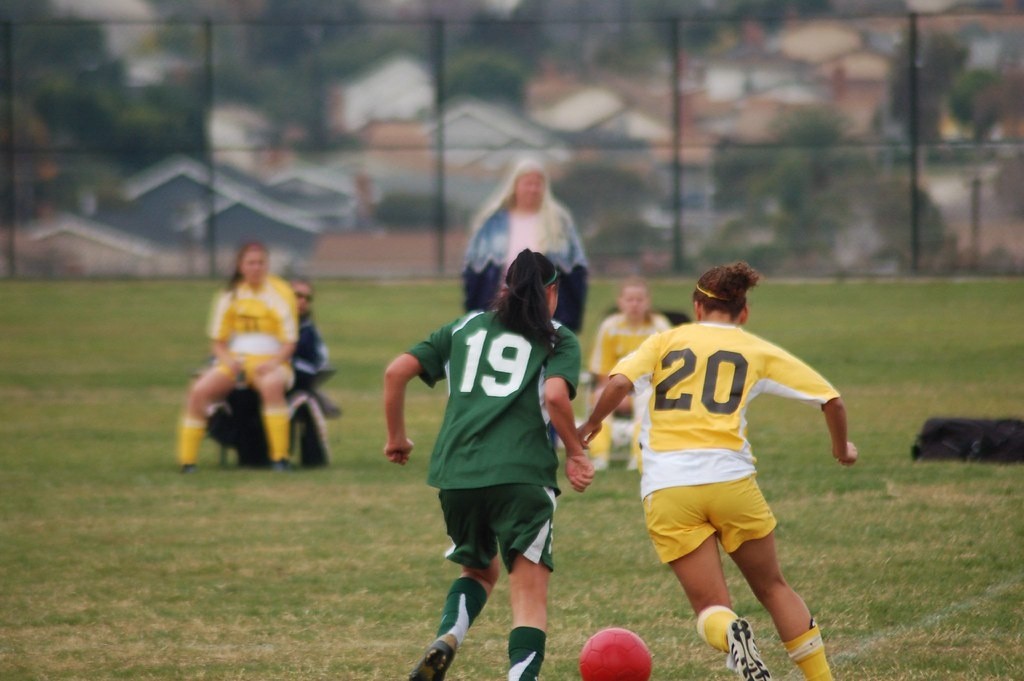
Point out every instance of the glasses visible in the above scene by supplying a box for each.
[294,291,312,304]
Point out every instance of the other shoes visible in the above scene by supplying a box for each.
[274,460,292,472]
[180,464,195,474]
[726,619,770,681]
[408,633,457,681]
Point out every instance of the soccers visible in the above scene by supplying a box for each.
[578,628,651,681]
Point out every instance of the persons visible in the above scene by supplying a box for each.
[462,157,588,334]
[176,243,329,474]
[576,260,858,681]
[587,275,673,471]
[384,248,595,681]
